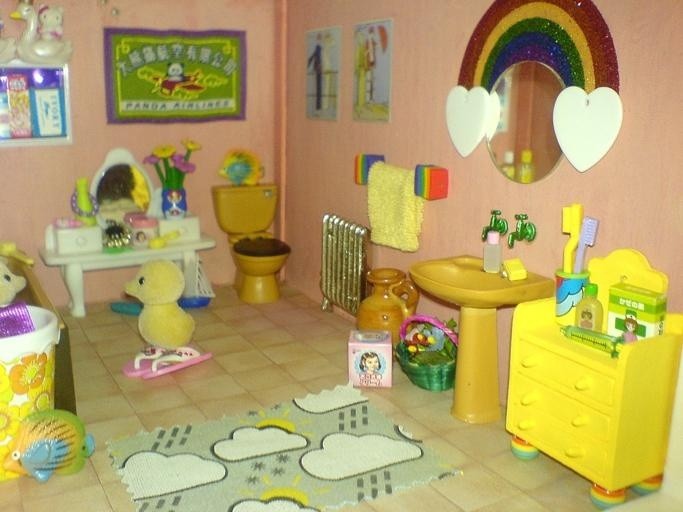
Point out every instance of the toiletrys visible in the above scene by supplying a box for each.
[484,231,502,273]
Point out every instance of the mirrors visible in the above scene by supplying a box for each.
[484,60,568,184]
[89,146,155,230]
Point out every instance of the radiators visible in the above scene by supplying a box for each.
[320,213,371,317]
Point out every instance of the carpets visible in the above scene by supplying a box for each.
[104,381,464,512]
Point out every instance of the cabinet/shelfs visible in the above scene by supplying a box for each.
[505,247,683,508]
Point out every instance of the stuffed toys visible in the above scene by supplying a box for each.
[123,261,194,349]
[0,256,27,308]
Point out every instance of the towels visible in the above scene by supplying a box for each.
[366,160,426,253]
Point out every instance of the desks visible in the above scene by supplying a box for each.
[39,236,217,317]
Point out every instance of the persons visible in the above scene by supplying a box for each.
[359,353,382,387]
[580,309,593,327]
[621,309,638,342]
